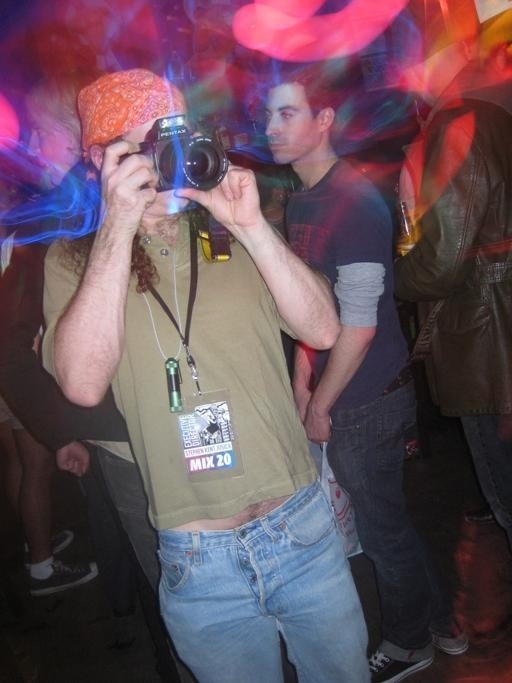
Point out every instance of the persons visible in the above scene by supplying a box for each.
[262,61,471,682]
[1,395,100,598]
[1,65,177,680]
[40,63,380,683]
[387,0,512,546]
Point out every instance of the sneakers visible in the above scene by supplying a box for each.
[366,647,436,683]
[426,621,472,655]
[26,560,99,599]
[19,528,75,562]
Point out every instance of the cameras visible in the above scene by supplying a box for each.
[119,114,229,193]
